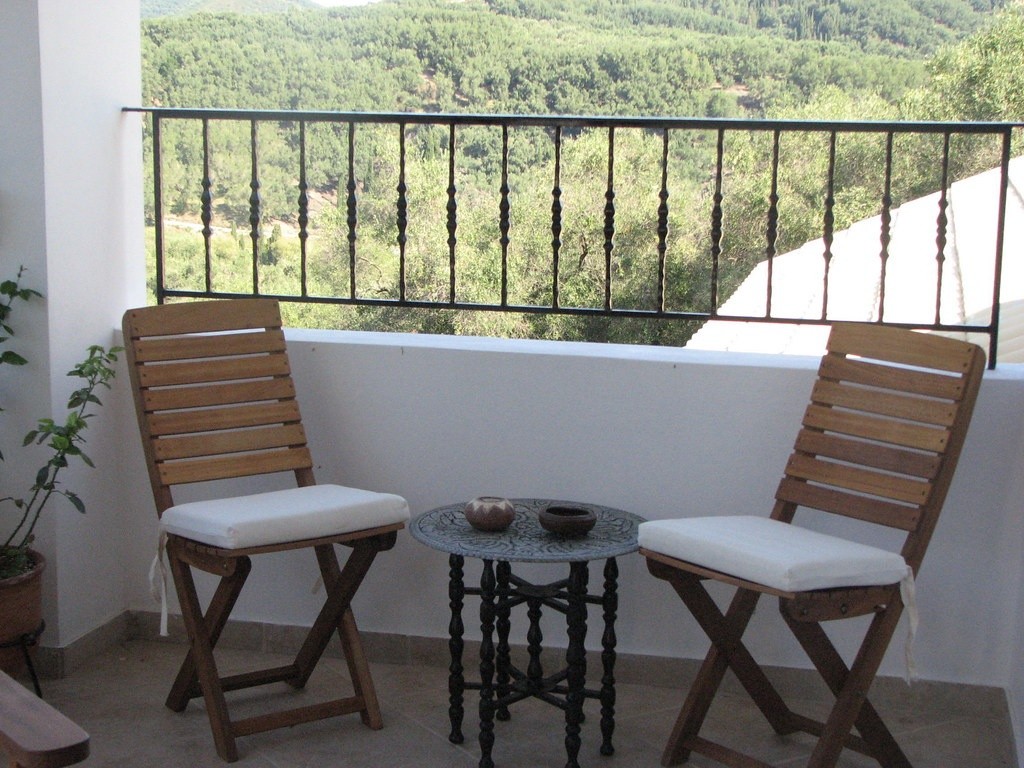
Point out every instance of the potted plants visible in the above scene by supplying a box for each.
[0,264,125,645]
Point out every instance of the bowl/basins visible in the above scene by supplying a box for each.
[539,506,597,535]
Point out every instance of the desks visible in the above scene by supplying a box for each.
[407,496,649,768]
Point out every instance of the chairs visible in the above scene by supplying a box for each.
[123,297,414,764]
[637,322,987,767]
